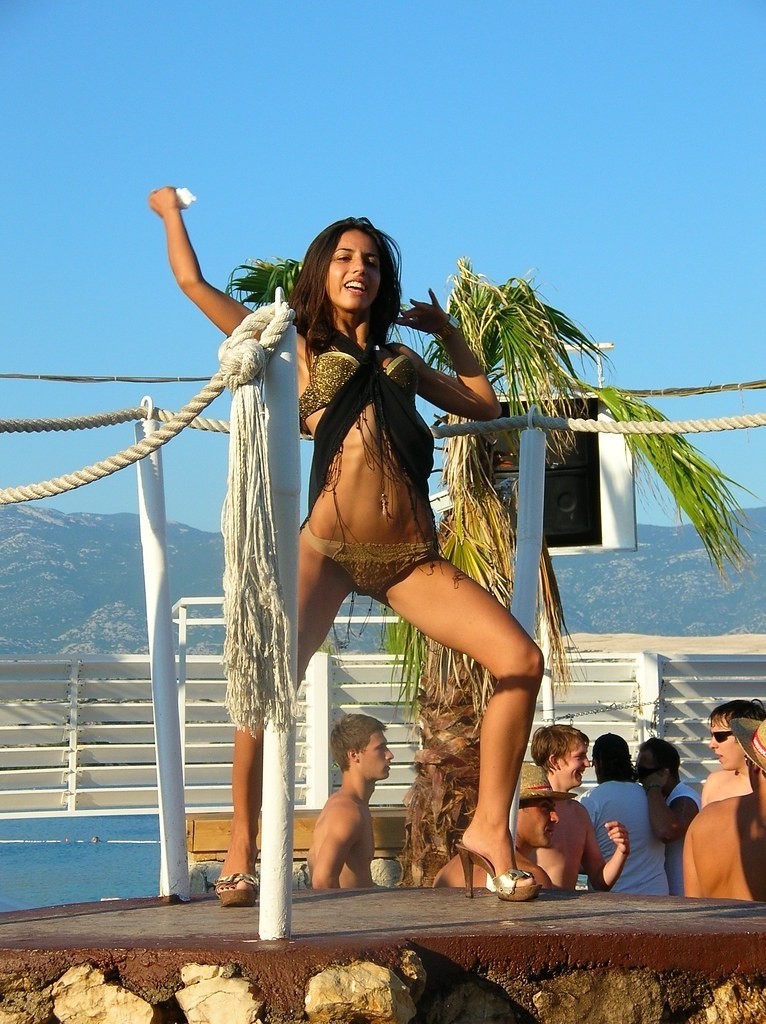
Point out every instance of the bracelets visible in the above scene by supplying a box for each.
[645,783,662,795]
[431,313,461,341]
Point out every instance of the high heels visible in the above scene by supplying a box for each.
[455,843,543,901]
[214,873,259,907]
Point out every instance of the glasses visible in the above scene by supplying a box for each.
[637,767,659,778]
[744,755,754,766]
[710,730,733,743]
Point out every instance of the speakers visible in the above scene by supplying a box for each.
[483,392,638,556]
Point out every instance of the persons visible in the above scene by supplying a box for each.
[577,733,669,895]
[432,763,579,889]
[682,718,766,902]
[527,724,631,892]
[700,699,766,810]
[305,713,394,890]
[148,185,545,910]
[635,737,703,896]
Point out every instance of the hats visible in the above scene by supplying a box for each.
[592,733,629,766]
[730,717,766,773]
[519,762,578,801]
